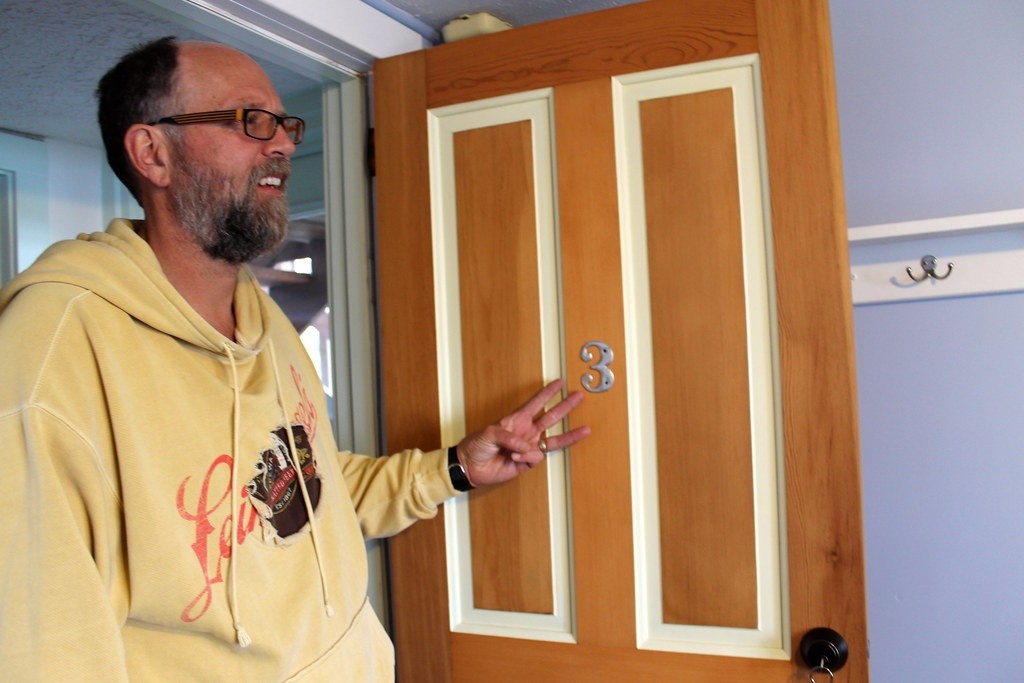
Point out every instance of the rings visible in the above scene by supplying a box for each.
[539,440,546,453]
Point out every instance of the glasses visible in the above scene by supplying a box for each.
[143,108,307,146]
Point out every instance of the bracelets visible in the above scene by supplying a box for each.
[448,445,476,491]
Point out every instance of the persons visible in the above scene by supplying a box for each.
[0,36,598,683]
